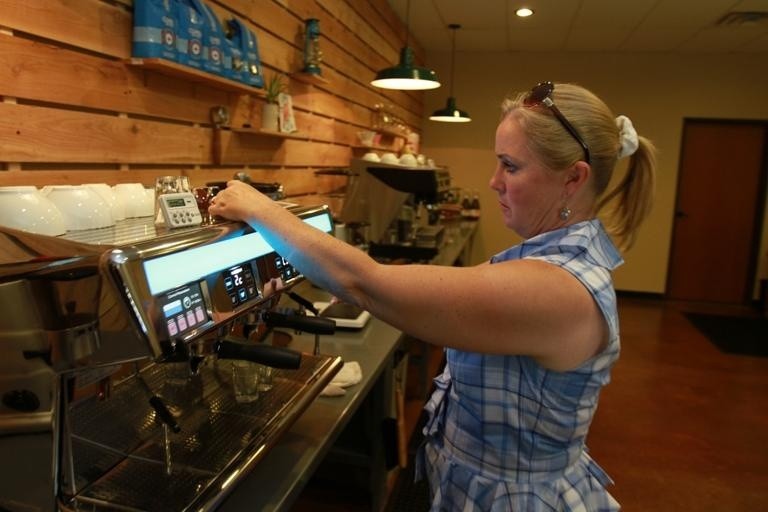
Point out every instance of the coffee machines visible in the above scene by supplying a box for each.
[339,159,451,263]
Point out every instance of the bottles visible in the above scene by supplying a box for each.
[458,190,481,222]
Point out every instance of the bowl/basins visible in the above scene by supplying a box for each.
[0,183,156,237]
[360,153,417,168]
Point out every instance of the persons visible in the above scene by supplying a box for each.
[203,79,659,511]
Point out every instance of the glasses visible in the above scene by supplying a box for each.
[523,80,590,165]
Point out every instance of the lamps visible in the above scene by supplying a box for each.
[370,0,442,92]
[429,24,473,123]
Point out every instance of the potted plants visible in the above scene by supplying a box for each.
[262,71,288,133]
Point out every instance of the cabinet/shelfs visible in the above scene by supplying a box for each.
[123,56,313,141]
[350,125,411,152]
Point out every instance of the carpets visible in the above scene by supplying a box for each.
[681,311,767,359]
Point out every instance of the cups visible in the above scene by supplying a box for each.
[233,357,275,403]
